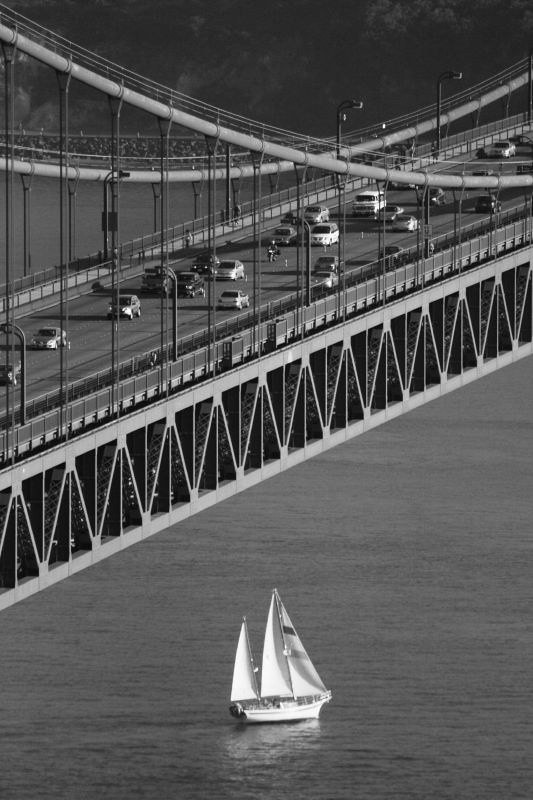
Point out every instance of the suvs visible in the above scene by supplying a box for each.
[141,272,170,298]
[189,254,221,275]
[106,294,141,321]
[474,195,502,214]
[172,272,205,298]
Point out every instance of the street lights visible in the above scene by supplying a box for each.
[436,71,463,152]
[103,167,130,260]
[336,98,363,160]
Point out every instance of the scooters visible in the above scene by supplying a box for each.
[266,247,280,263]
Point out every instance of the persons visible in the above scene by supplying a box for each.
[268,241,278,259]
[231,204,241,226]
[273,698,277,709]
[363,140,437,170]
[149,352,157,369]
[184,230,192,248]
[429,242,434,255]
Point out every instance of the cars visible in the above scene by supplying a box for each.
[0,363,18,386]
[268,227,297,245]
[375,205,404,223]
[396,182,415,190]
[487,141,517,159]
[215,260,245,281]
[31,327,67,351]
[216,290,250,310]
[303,205,330,225]
[313,256,346,274]
[391,215,422,233]
[418,187,447,207]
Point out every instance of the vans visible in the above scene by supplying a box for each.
[309,222,340,247]
[352,190,387,218]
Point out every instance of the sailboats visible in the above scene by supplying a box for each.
[228,587,333,723]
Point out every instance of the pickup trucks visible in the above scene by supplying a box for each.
[509,135,533,146]
